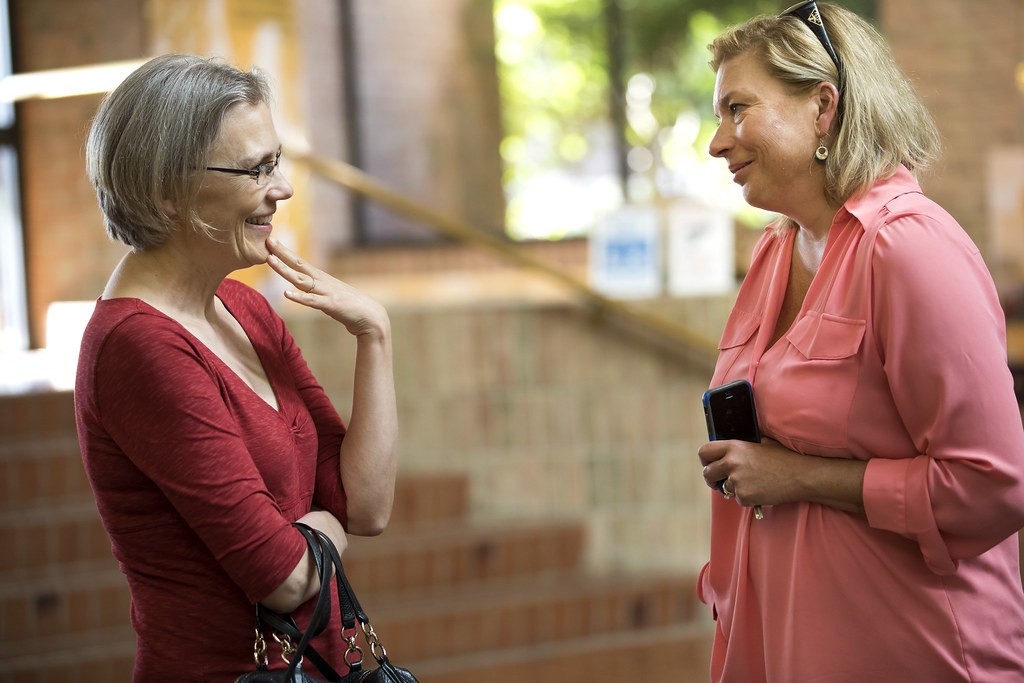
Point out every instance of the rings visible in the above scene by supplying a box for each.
[307,278,316,293]
[722,480,735,499]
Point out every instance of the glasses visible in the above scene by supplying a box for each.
[194,148,281,186]
[779,0,847,125]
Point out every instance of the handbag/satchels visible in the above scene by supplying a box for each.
[233,522,419,683]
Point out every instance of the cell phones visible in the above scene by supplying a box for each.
[702,380,761,495]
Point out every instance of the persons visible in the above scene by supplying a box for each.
[74,53,401,682]
[697,0,1023,683]
